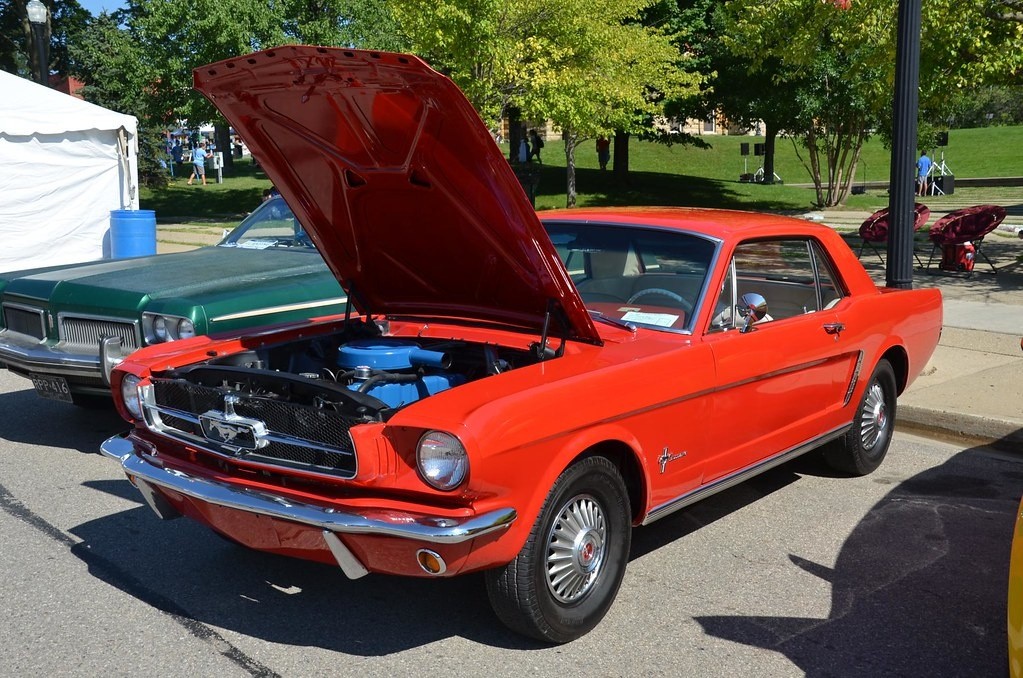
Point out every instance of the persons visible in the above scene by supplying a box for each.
[529,130,544,165]
[187,143,213,185]
[596,135,610,174]
[916,150,931,197]
[171,140,183,166]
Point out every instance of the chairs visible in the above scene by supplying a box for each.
[576,244,642,307]
[632,243,714,319]
[929,205,1005,280]
[856,202,930,267]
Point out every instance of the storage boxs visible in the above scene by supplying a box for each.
[110,210,156,261]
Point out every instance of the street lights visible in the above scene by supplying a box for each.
[23,0,50,87]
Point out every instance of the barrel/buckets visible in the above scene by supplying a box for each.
[109,208,159,261]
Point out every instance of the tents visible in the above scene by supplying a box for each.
[1,68,138,274]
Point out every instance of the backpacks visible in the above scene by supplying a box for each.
[534,135,546,149]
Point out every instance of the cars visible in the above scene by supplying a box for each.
[94,39,944,643]
[0,192,594,428]
